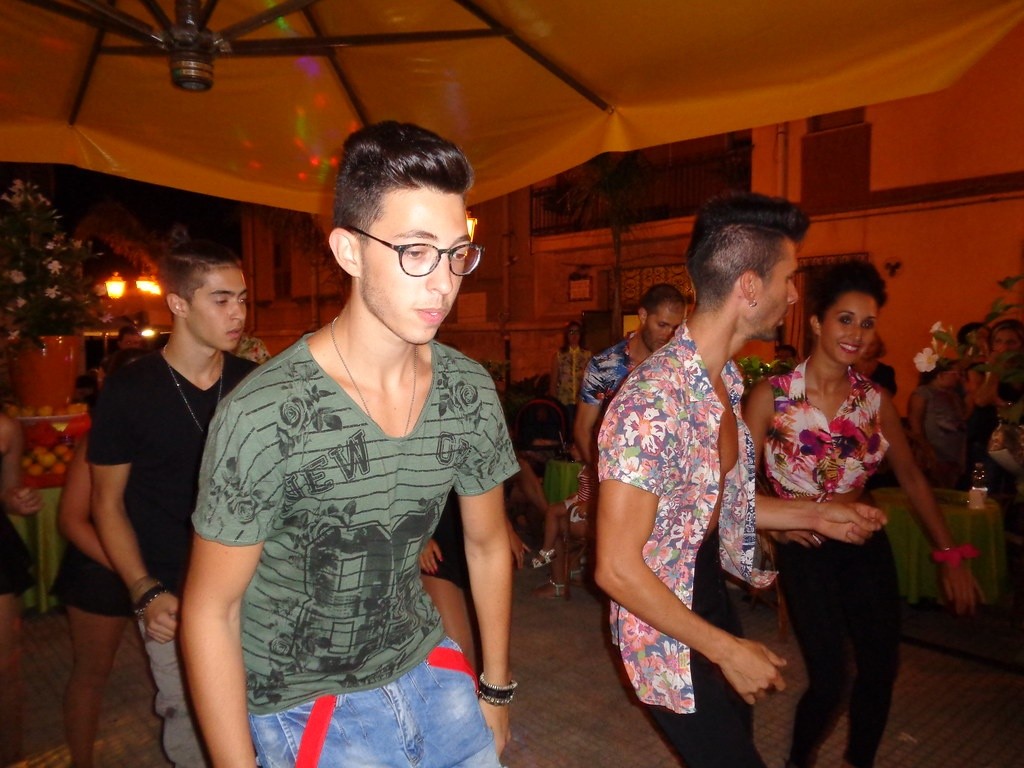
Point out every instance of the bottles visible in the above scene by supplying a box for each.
[973,462,986,489]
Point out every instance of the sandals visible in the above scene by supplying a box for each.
[568,568,590,589]
[526,548,557,570]
[537,576,569,599]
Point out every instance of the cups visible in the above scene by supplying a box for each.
[555,442,569,459]
[968,488,986,511]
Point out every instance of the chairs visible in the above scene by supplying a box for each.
[565,496,598,594]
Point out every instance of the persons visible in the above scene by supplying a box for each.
[770,319,1024,612]
[591,189,886,768]
[745,258,988,768]
[0,320,271,768]
[419,282,685,681]
[179,121,523,768]
[85,238,263,768]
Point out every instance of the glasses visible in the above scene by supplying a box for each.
[344,225,486,278]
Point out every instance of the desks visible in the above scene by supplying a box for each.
[871,487,1007,605]
[541,458,585,509]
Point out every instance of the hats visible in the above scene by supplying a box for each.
[988,307,1024,328]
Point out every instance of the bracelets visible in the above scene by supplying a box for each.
[129,574,164,605]
[475,673,517,703]
[134,585,168,622]
[927,541,979,566]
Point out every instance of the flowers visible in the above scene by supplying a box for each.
[912,323,958,376]
[0,175,113,358]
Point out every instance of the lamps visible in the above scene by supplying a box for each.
[103,270,128,301]
[136,271,165,299]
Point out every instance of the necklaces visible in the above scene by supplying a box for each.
[332,316,417,437]
[164,342,223,437]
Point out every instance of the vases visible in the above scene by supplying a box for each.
[7,334,82,410]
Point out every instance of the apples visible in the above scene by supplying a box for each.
[2,402,89,417]
[18,445,75,476]
